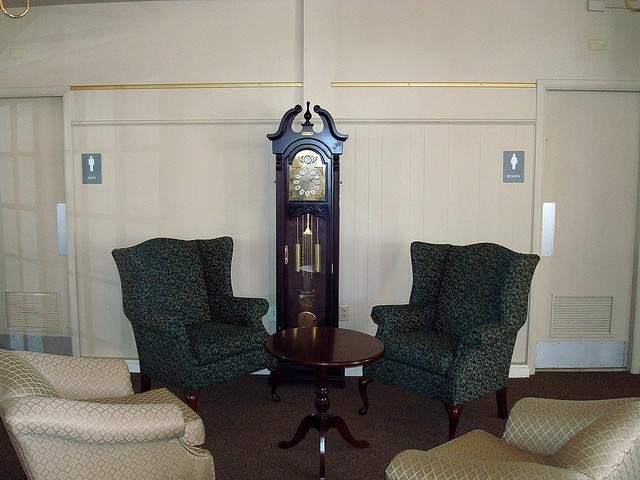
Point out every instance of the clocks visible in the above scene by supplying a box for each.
[287,166,326,202]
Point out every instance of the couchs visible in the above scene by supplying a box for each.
[358,241,540,441]
[111,236,281,411]
[1,348,216,480]
[385,397,640,480]
